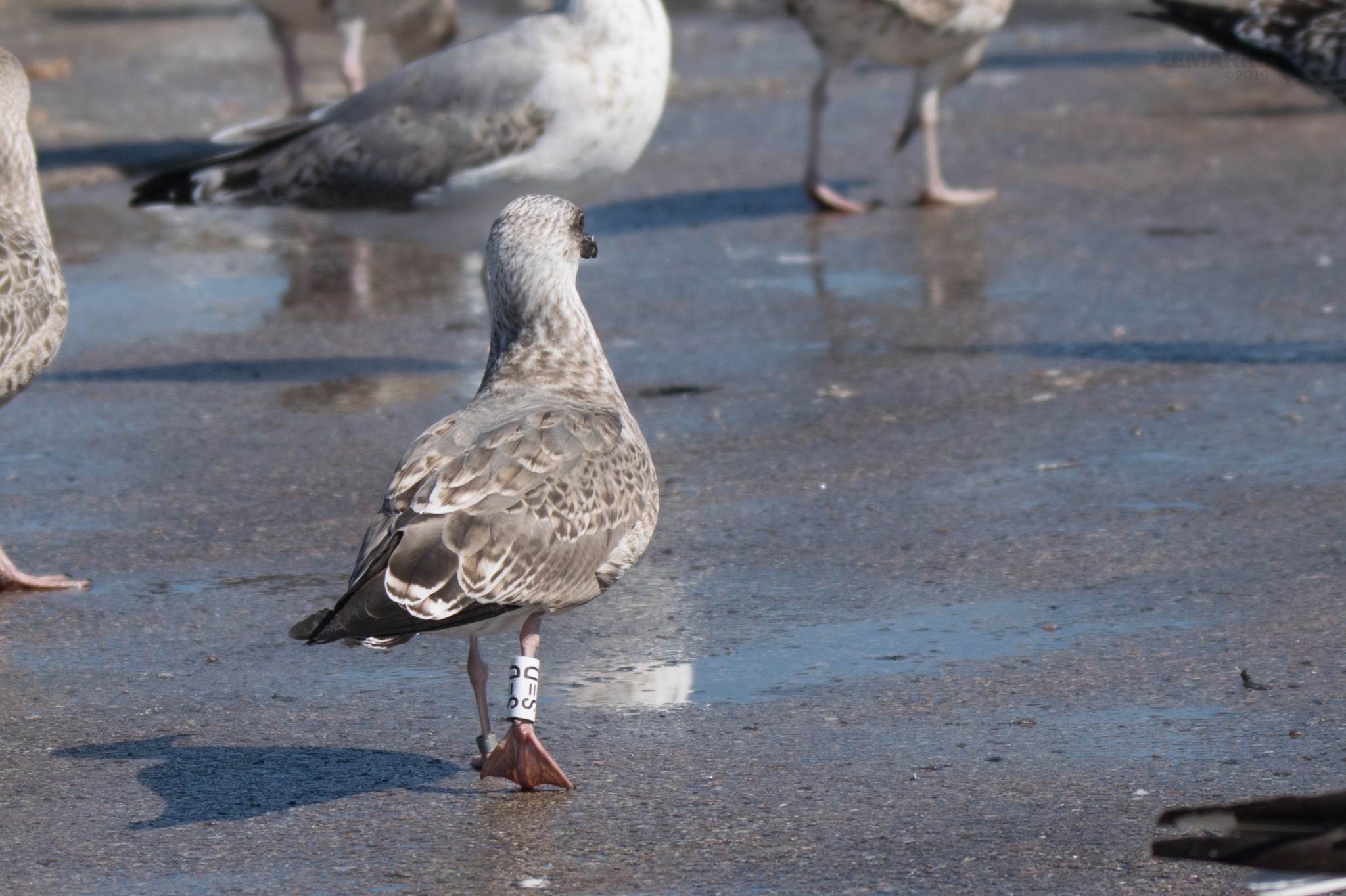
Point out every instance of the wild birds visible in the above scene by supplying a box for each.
[1126,0,1346,101]
[784,0,1016,215]
[0,47,91,591]
[124,0,671,217]
[289,196,659,791]
